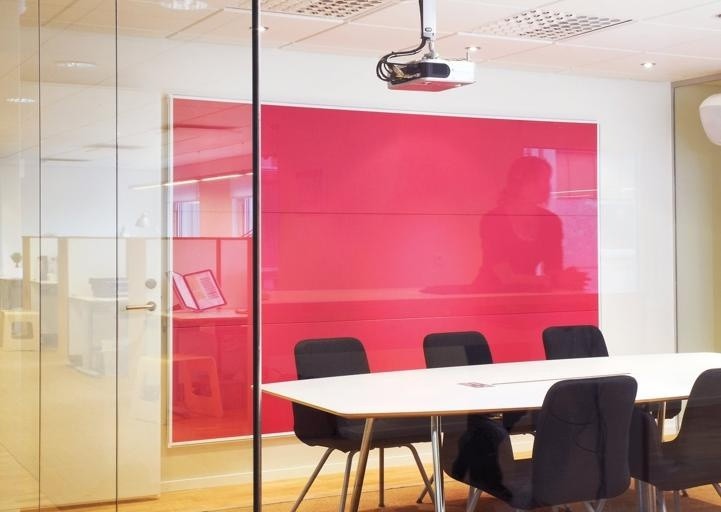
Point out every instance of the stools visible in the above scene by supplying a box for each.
[130,353,224,424]
[1,307,39,352]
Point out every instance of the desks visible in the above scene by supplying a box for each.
[161,308,247,410]
[68,296,129,377]
[250,352,721,512]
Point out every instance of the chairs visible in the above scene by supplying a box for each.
[636,368,721,512]
[541,325,688,500]
[466,377,635,511]
[417,331,534,503]
[290,338,432,512]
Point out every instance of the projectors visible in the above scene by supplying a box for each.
[376,28,476,93]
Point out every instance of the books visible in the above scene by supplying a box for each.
[173,269,228,311]
